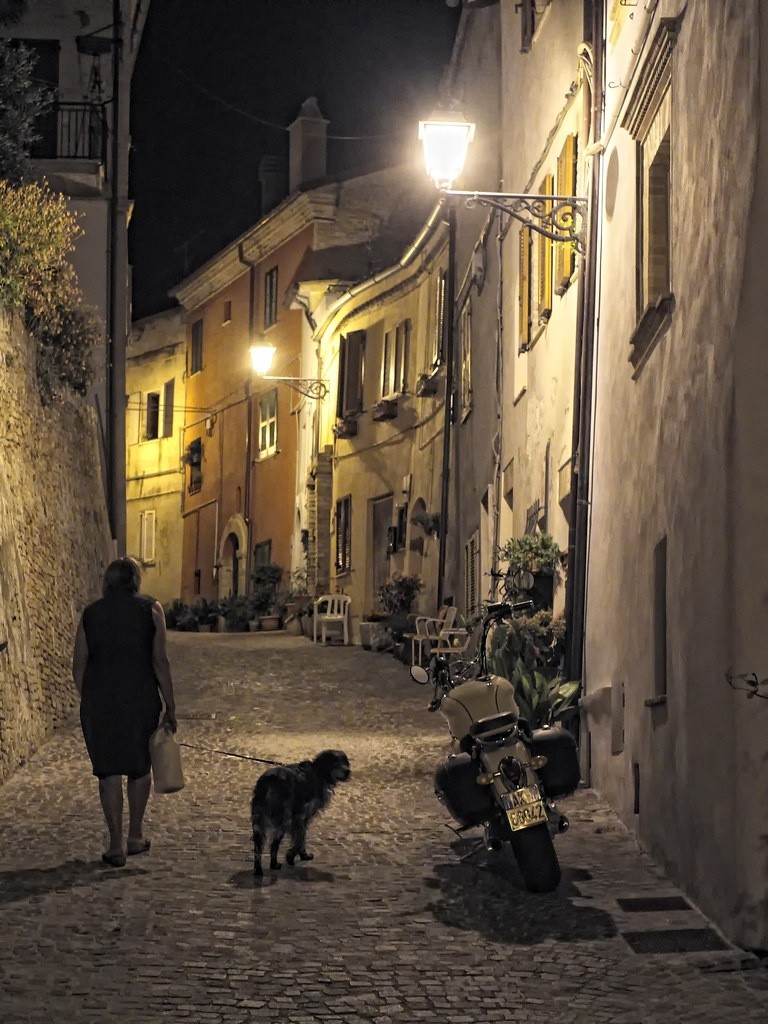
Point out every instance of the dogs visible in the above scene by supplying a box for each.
[250,749,354,876]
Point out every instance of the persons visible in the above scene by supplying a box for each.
[72,557,178,867]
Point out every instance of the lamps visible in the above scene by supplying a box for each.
[418,109,588,259]
[249,331,329,402]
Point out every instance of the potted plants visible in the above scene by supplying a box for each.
[197,613,213,632]
[259,592,280,631]
[292,565,313,610]
[284,571,295,616]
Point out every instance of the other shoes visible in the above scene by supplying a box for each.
[128,839,151,853]
[102,851,126,867]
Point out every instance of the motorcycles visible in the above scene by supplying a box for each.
[408,569,581,896]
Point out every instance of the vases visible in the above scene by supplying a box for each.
[249,621,259,633]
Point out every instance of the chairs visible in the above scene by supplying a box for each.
[412,606,457,668]
[431,626,475,658]
[313,593,352,645]
[403,605,449,667]
